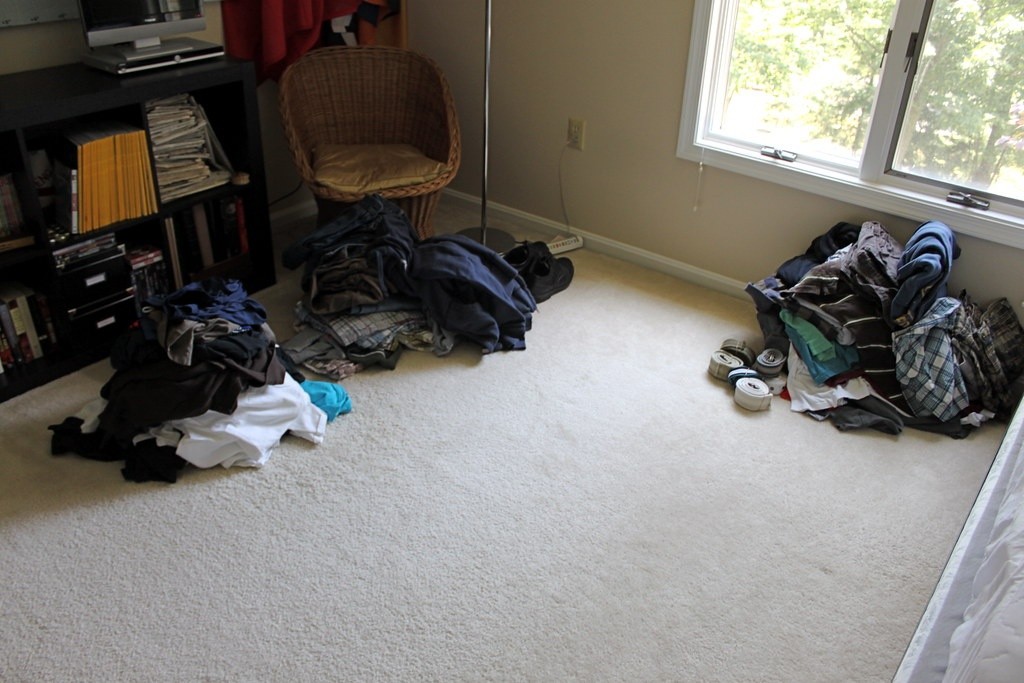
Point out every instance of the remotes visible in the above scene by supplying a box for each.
[547,236,583,254]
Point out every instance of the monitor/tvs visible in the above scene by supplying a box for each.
[78,0,207,61]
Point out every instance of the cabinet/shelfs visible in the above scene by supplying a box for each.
[0,57,280,407]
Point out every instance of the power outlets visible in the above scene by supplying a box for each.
[565,117,586,152]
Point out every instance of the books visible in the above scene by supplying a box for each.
[145,92,232,202]
[163,193,249,290]
[49,125,159,234]
[0,283,58,375]
[53,233,116,269]
[124,244,176,310]
[0,173,24,237]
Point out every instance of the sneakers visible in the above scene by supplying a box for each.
[499,241,551,269]
[518,257,574,303]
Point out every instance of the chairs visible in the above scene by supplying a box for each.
[277,44,466,248]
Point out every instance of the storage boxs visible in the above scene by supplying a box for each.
[51,243,139,359]
